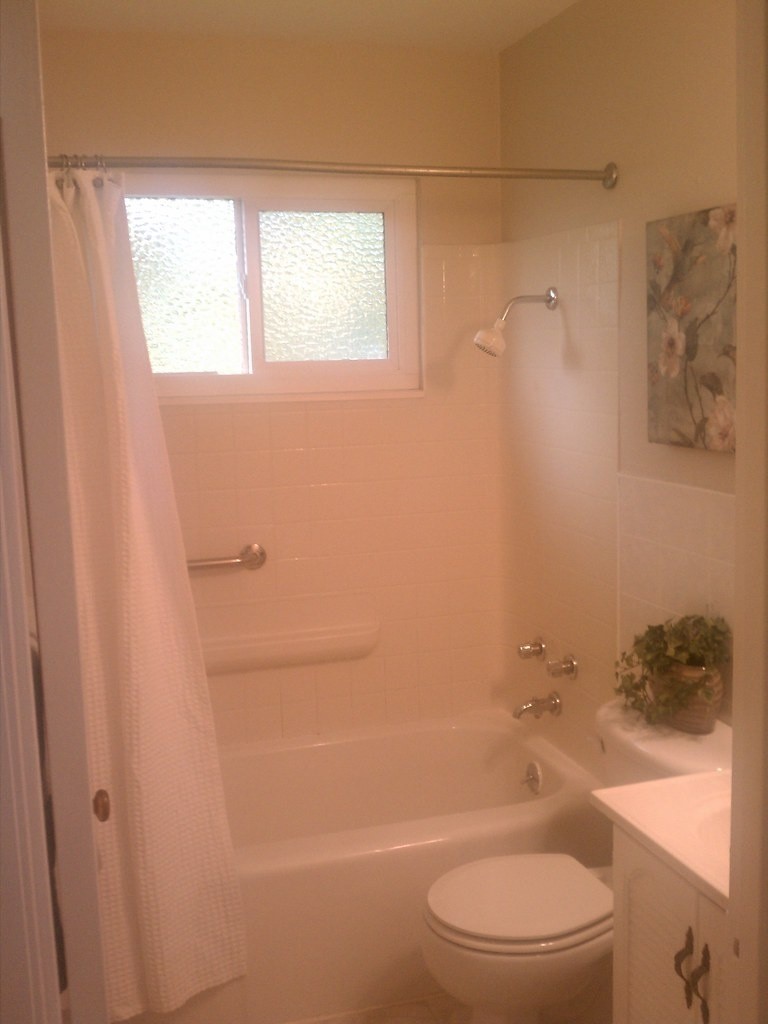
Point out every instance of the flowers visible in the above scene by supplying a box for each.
[706,391,737,451]
[657,315,686,380]
[709,205,738,252]
[616,612,734,725]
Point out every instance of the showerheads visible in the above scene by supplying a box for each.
[472,317,512,361]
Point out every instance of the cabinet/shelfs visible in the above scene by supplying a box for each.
[590,769,732,1024]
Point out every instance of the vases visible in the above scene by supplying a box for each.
[669,668,724,734]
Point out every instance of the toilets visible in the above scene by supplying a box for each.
[416,687,736,1024]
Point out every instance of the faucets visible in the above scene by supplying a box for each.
[509,692,565,727]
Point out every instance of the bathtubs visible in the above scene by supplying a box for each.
[132,702,614,1023]
